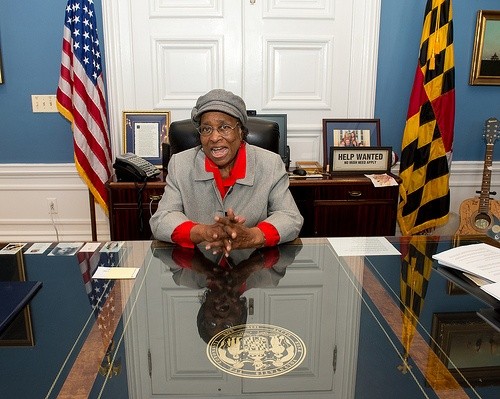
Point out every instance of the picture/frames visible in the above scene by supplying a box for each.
[322,119,381,170]
[330,146,392,174]
[448,235,500,296]
[425,311,500,389]
[0,243,34,347]
[469,10,500,85]
[296,161,323,173]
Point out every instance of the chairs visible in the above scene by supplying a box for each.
[0,234,500,399]
[169,118,280,155]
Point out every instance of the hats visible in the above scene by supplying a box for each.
[191,89,249,125]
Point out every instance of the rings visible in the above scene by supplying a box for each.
[228,240,232,243]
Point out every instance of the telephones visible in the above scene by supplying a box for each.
[113,152,161,181]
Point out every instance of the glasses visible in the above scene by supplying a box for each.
[194,122,241,136]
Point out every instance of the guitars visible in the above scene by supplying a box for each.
[453,118,500,235]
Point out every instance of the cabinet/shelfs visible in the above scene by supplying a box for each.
[106,168,403,241]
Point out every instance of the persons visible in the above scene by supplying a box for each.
[149,89,304,258]
[151,238,303,349]
[341,132,357,146]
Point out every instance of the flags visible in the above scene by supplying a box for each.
[56,0,113,216]
[397,0,456,237]
[400,237,440,359]
[76,242,119,354]
